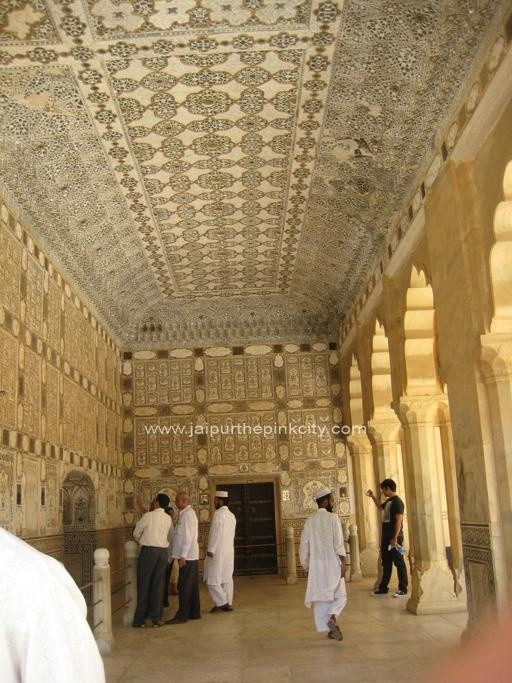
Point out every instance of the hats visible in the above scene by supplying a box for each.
[215,490,229,497]
[315,489,331,500]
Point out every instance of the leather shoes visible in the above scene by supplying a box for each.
[210,603,233,613]
[327,614,343,641]
[139,615,201,627]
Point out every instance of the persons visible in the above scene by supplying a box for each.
[298,486,347,642]
[130,490,238,628]
[0,524,104,683]
[405,605,512,683]
[365,479,409,599]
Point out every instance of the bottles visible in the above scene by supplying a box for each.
[393,543,407,555]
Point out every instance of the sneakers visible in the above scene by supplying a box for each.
[371,590,389,595]
[392,591,407,597]
